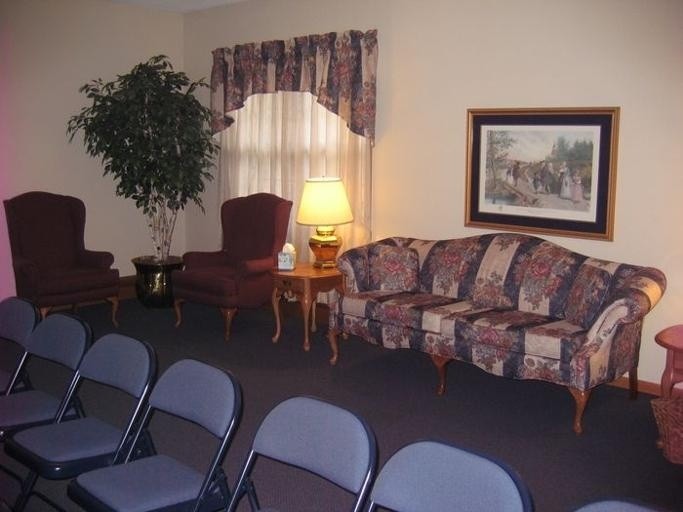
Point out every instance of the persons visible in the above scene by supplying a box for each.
[503,157,584,203]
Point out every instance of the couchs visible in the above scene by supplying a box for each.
[326,233,667,436]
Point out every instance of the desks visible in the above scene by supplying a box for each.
[269,264,345,351]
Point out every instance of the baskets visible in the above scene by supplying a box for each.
[652,397,682,464]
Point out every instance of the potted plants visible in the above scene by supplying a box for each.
[64,55,223,308]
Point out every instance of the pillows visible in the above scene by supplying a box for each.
[367,242,419,295]
[563,264,613,331]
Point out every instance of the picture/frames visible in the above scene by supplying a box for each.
[463,105,620,242]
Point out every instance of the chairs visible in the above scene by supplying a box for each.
[171,192,292,344]
[3,190,120,327]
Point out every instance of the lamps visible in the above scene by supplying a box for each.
[295,176,354,268]
